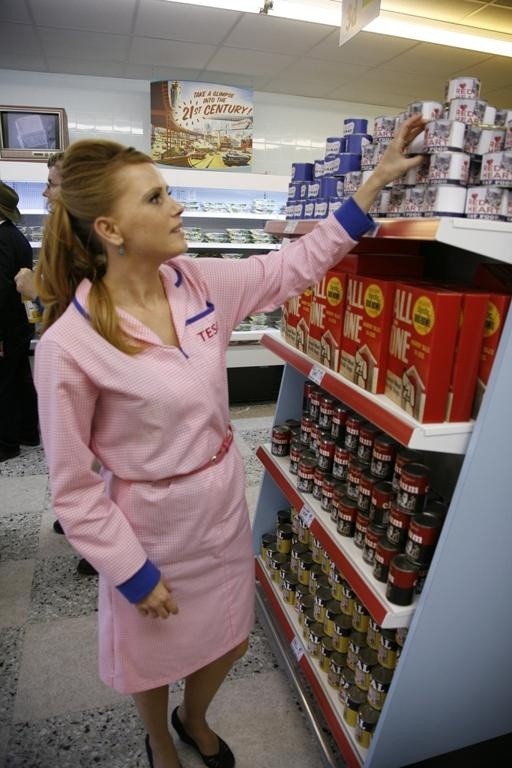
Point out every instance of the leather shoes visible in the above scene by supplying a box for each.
[172,706,234,768]
[145,734,181,767]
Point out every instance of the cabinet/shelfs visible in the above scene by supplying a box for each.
[0,161,292,404]
[245,215,512,768]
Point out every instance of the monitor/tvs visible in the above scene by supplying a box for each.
[0,105,70,163]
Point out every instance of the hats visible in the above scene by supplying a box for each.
[0,181,21,221]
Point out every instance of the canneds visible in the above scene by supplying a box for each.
[286,77,512,222]
[271,381,441,607]
[262,505,409,748]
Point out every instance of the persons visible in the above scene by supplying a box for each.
[15,152,99,576]
[0,181,39,462]
[33,113,427,768]
